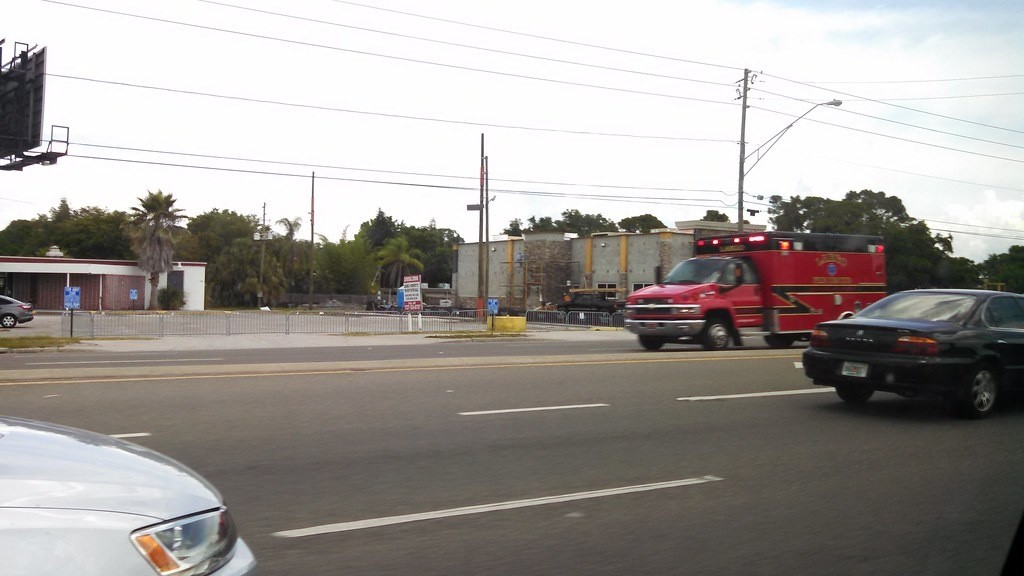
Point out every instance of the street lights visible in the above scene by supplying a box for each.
[739,100,844,235]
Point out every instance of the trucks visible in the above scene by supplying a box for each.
[624,231,887,351]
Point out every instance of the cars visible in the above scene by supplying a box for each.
[801,288,1024,420]
[0,415,259,576]
[0,294,34,328]
[557,293,617,324]
